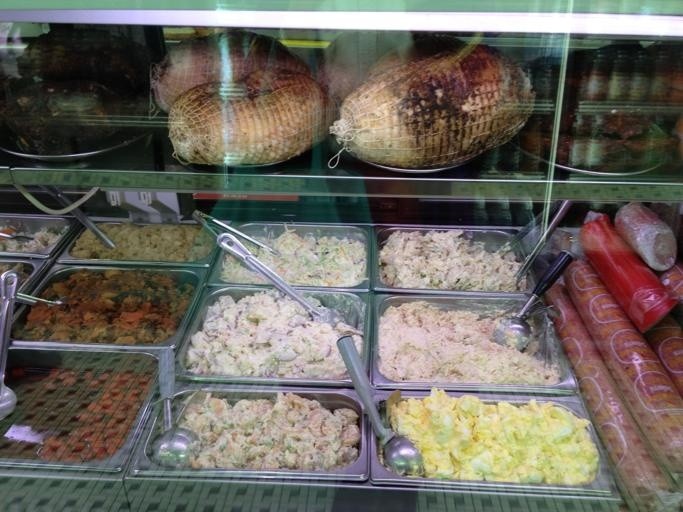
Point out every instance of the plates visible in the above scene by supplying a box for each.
[0,130,154,162]
[510,126,671,177]
[345,151,483,175]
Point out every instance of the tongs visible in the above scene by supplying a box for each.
[494,200,574,286]
[189,209,280,259]
